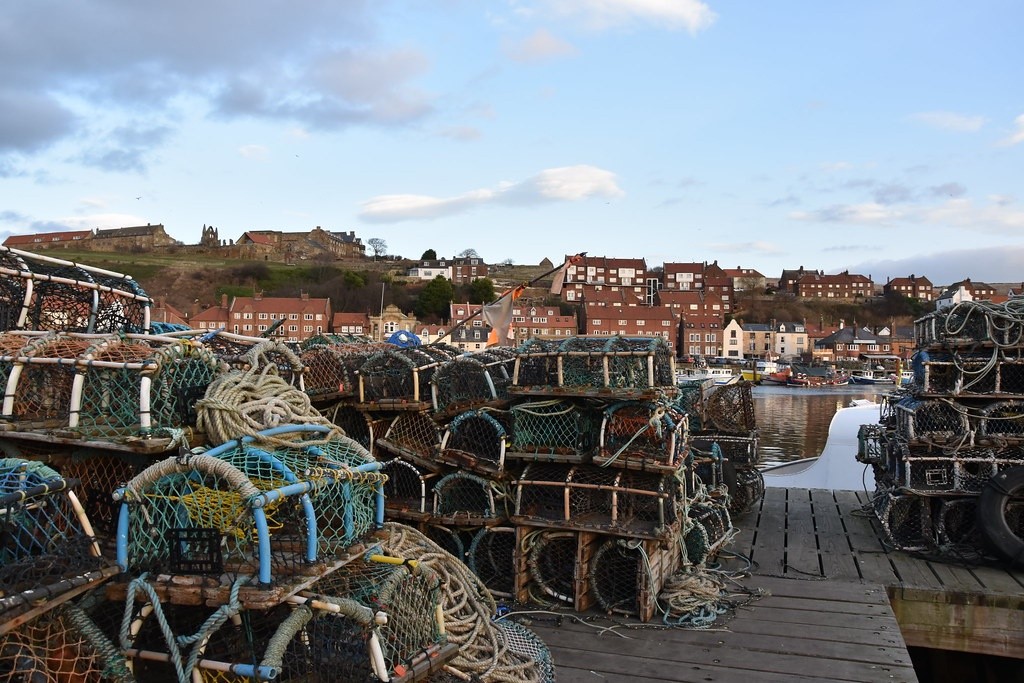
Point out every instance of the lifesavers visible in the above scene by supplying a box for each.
[797,373,808,380]
[977,465,1024,568]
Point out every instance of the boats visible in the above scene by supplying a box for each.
[769,369,822,385]
[901,372,914,384]
[786,371,817,386]
[741,335,778,383]
[848,370,894,384]
[677,358,743,387]
